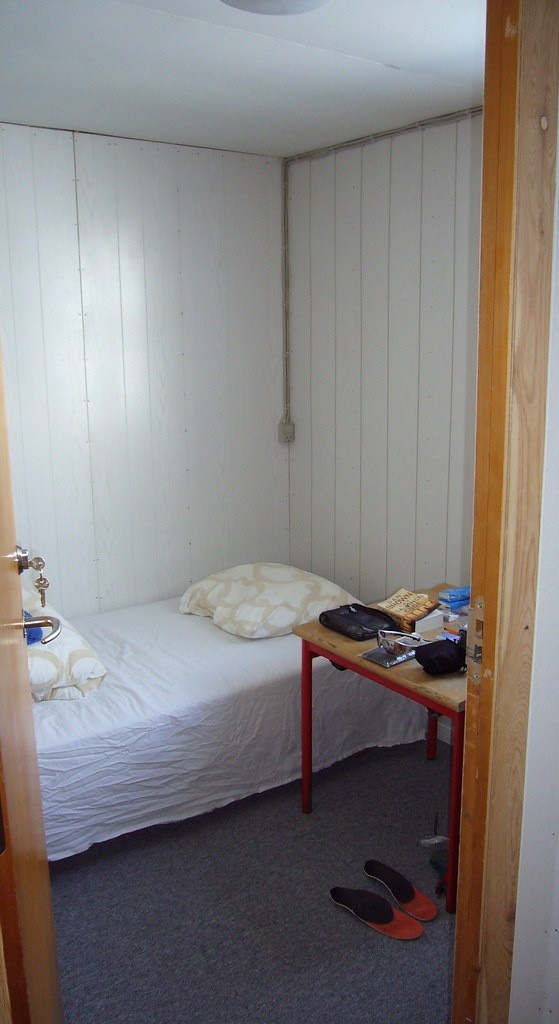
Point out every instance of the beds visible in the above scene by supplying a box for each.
[31,596,427,863]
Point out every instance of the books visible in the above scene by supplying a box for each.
[376,587,445,635]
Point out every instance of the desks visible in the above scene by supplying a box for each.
[292,582,467,915]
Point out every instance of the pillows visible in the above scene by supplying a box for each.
[179,563,365,641]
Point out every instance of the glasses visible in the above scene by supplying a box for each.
[377,629,420,659]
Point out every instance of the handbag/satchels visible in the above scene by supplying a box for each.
[318,602,398,640]
